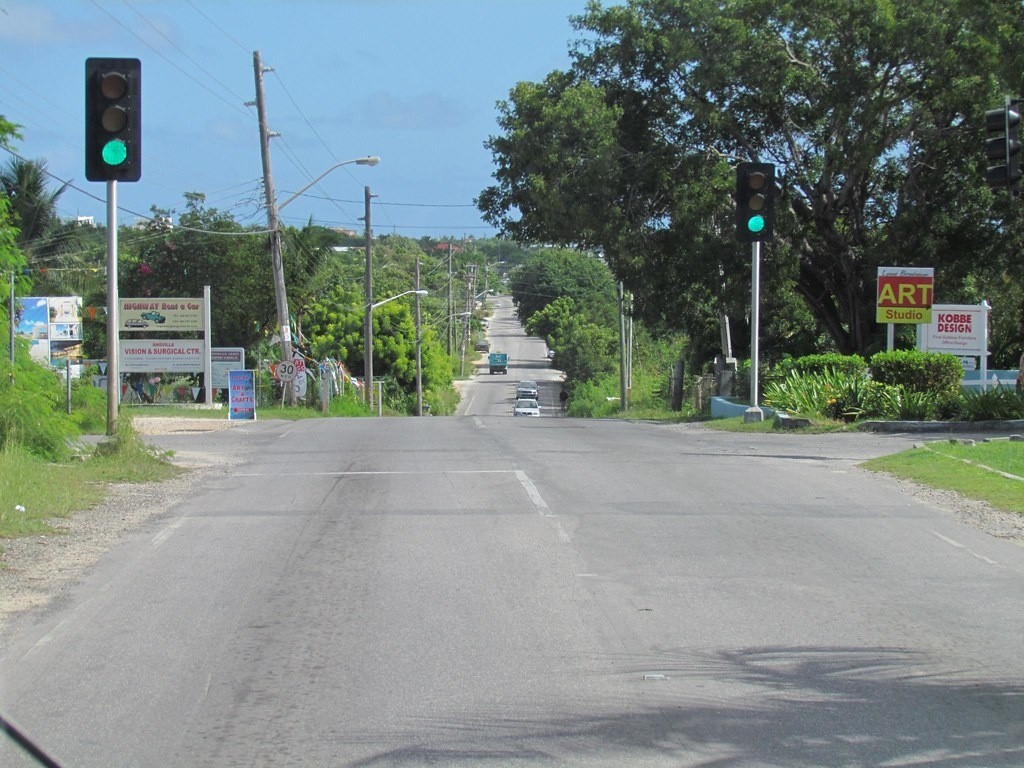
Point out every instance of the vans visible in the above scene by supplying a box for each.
[124,319,149,328]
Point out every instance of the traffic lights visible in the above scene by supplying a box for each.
[85,57,142,183]
[984,96,1024,204]
[736,161,775,240]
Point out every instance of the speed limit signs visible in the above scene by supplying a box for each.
[277,360,297,382]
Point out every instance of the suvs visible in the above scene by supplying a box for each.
[514,380,542,400]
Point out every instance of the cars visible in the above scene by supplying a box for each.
[474,339,491,353]
[141,310,165,323]
[512,397,543,417]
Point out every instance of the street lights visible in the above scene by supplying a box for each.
[365,289,429,413]
[416,312,471,417]
[484,261,505,296]
[471,288,494,313]
[267,155,381,405]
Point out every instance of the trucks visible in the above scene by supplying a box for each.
[487,352,511,375]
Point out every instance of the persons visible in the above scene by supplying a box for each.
[560,390,568,412]
